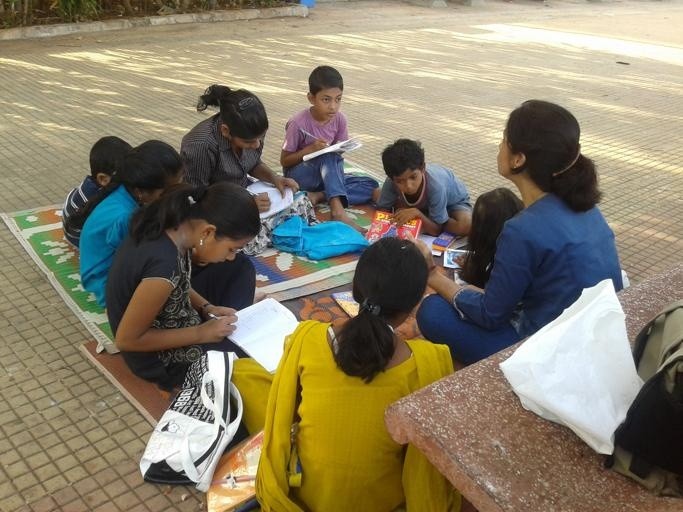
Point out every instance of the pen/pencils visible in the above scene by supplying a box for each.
[245,189,260,196]
[299,127,331,148]
[207,312,221,320]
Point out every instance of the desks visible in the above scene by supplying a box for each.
[380,259,681,511]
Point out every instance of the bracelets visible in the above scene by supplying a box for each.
[198,302,211,316]
[428,264,437,272]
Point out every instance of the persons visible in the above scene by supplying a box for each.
[453,188,525,289]
[61,136,134,248]
[105,181,262,392]
[373,138,474,237]
[232,237,463,512]
[79,139,187,311]
[280,66,379,237]
[180,84,300,214]
[404,99,623,364]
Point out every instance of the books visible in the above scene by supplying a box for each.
[330,291,360,319]
[206,429,268,512]
[227,297,298,376]
[303,136,364,161]
[245,178,294,220]
[366,210,422,246]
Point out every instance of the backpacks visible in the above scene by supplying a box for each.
[607,304,683,499]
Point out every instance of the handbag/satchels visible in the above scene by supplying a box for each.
[137,346,244,493]
[500,279,646,456]
[271,215,371,261]
